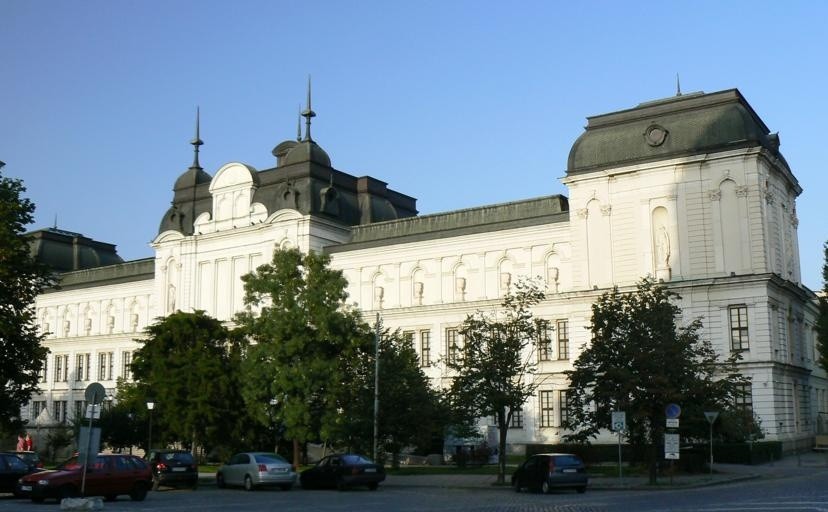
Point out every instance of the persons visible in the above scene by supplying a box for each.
[24,434,33,451]
[16,435,26,452]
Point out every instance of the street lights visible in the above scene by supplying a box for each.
[146,400,155,452]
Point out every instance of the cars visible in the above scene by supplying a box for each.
[16,450,43,468]
[512,453,588,494]
[216,452,296,492]
[299,453,385,492]
[142,449,199,491]
[1,453,49,499]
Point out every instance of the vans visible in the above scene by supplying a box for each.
[18,453,154,504]
